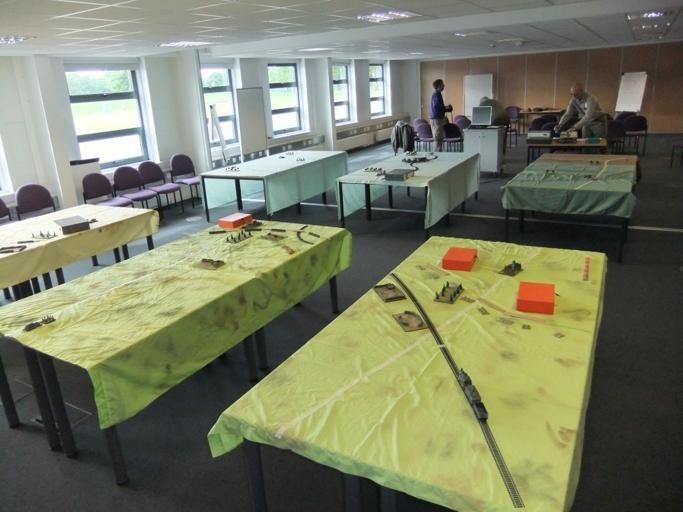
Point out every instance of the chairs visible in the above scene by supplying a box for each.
[0,152,202,236]
[670,141,683,168]
[413,106,647,164]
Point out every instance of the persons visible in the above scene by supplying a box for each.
[478,96,509,155]
[427,79,453,151]
[553,82,611,155]
